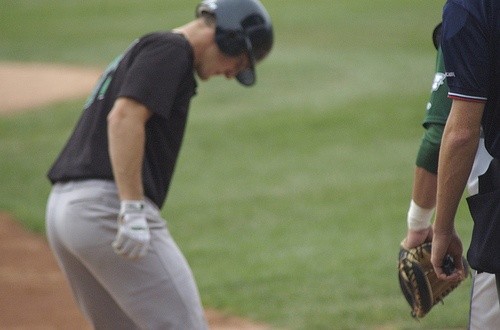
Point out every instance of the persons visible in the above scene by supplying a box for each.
[45,1,275,330]
[405,0,500,330]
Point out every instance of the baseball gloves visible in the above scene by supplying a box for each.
[398,240,473,323]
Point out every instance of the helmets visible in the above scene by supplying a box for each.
[197,0,273,87]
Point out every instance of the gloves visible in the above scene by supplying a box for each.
[112,200,150,261]
